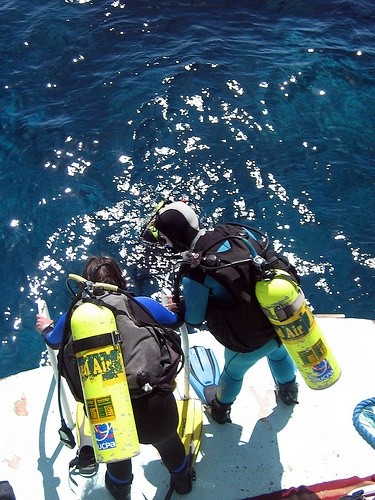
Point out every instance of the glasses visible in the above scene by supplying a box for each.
[156,228,167,246]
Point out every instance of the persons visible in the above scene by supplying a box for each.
[35,255,203,500]
[141,199,301,425]
